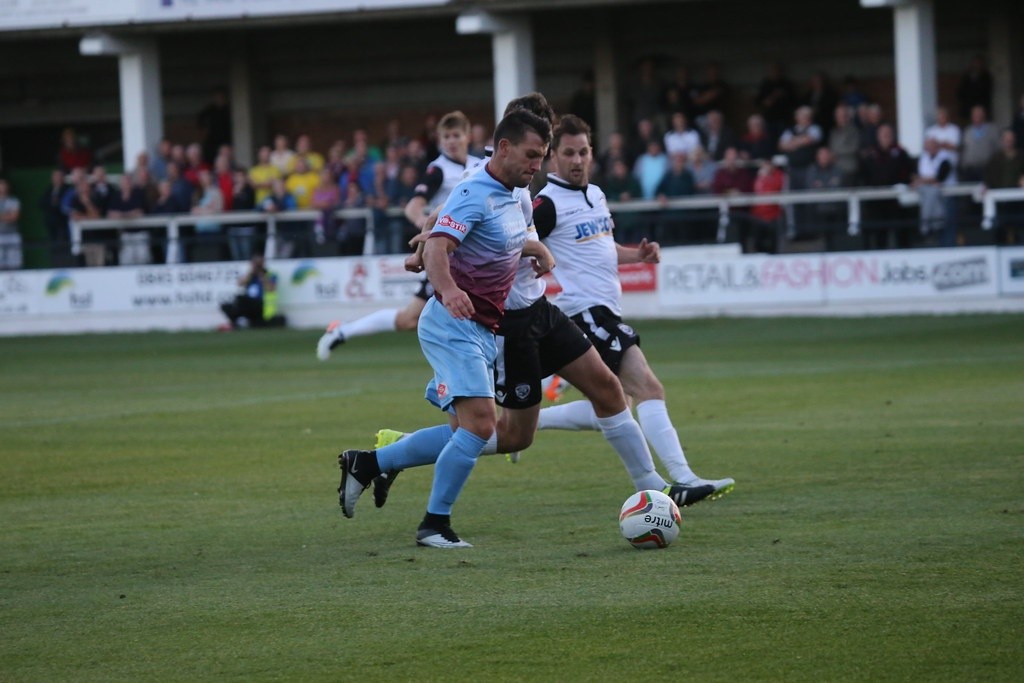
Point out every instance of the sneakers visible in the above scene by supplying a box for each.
[375,428,403,507]
[660,483,715,508]
[339,450,366,517]
[417,521,472,548]
[679,477,735,498]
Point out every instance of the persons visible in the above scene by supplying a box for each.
[373,91,713,508]
[338,105,554,549]
[1,60,1021,270]
[316,111,480,360]
[409,113,735,506]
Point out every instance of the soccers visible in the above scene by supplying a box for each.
[618,490,683,548]
